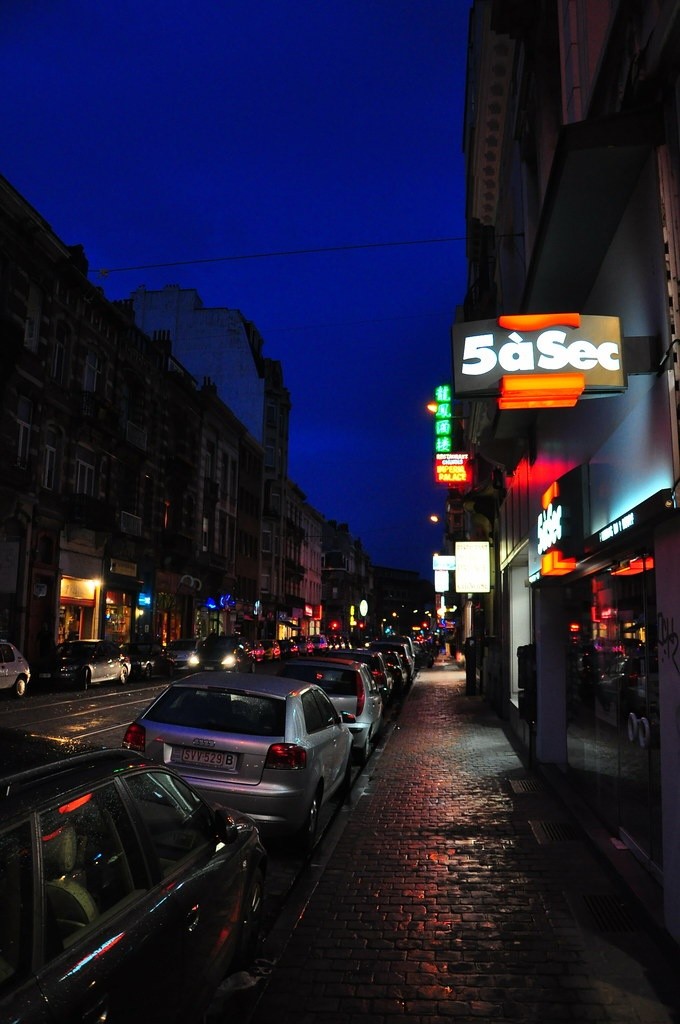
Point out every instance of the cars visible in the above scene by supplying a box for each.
[30,639,132,690]
[0,639,30,699]
[0,727,268,1024]
[119,671,355,862]
[276,634,415,769]
[119,642,166,682]
[165,634,327,674]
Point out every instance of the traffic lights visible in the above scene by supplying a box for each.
[332,622,338,628]
[423,622,427,628]
[385,627,391,634]
[359,623,365,629]
